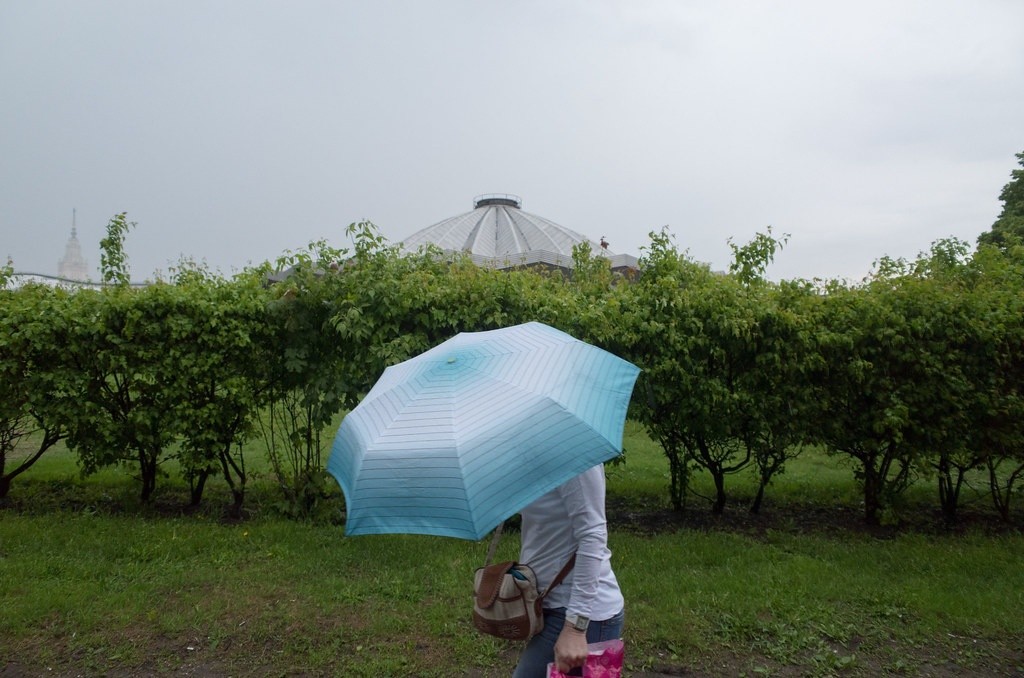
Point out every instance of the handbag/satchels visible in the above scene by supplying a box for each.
[472,562,547,642]
[547,637,624,678]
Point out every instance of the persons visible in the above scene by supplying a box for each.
[512,464,624,678]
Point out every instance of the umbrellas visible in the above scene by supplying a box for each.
[324,320,643,541]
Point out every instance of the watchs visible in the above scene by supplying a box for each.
[564,614,590,632]
